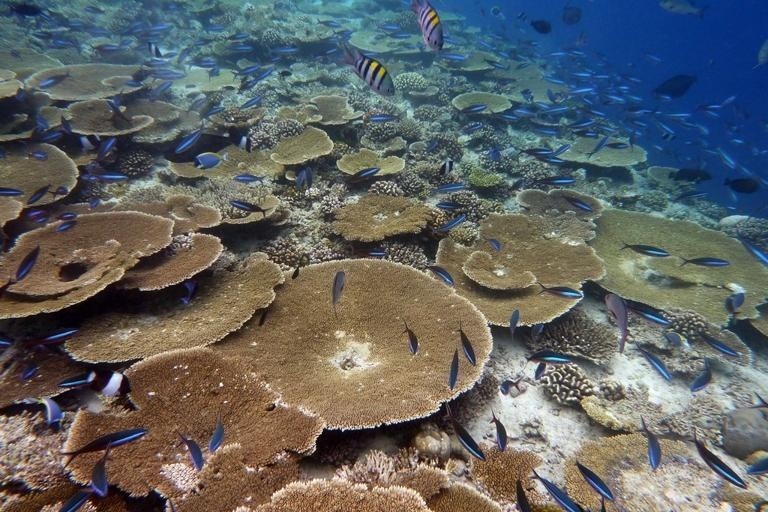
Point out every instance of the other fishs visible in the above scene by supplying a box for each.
[0,0,768,512]
